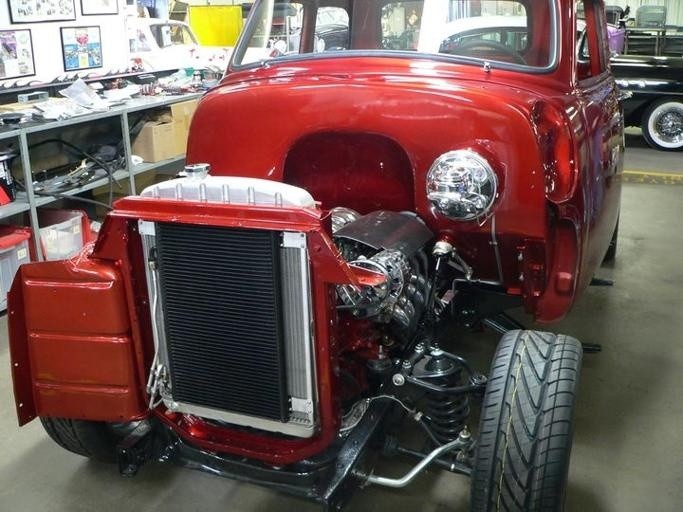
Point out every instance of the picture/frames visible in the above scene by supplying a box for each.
[0,0,121,82]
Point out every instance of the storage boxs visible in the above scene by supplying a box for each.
[128,99,199,164]
[0,208,84,314]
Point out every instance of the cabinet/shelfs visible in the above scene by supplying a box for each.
[0,92,206,317]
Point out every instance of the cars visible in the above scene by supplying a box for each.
[438,6,682,150]
[0,1,627,512]
[269,7,418,54]
[128,13,271,68]
